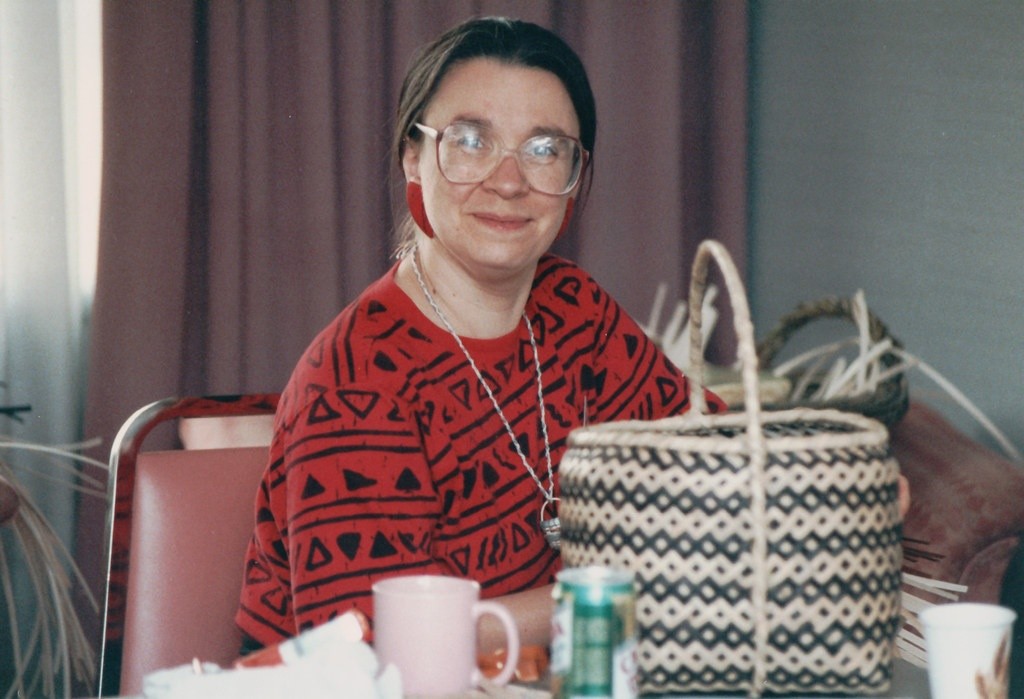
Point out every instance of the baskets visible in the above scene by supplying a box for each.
[558,240,899,699]
[713,294,910,443]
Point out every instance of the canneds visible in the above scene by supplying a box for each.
[549,568,639,699]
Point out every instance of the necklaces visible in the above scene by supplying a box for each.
[410,231,566,554]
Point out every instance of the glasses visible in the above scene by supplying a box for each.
[414,122,590,197]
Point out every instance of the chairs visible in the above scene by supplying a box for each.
[97,391,285,697]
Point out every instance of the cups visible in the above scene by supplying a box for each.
[372,575,520,699]
[919,602,1017,699]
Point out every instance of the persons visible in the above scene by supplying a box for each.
[235,15,728,673]
